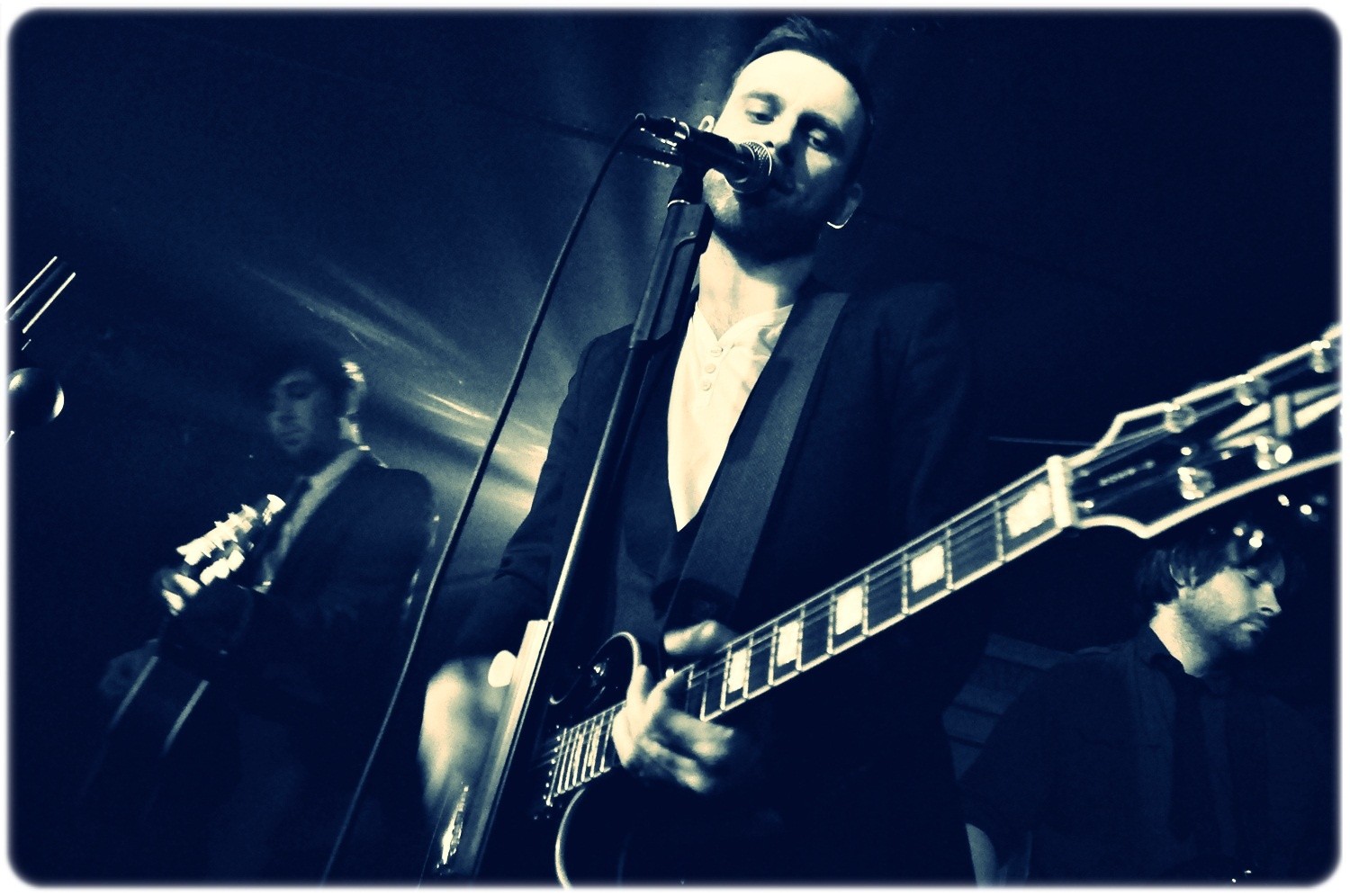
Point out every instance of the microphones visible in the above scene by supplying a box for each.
[637,113,772,192]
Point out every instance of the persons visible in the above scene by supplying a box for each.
[412,18,999,886]
[144,334,438,815]
[960,501,1318,889]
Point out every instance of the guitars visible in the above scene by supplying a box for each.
[421,322,1342,889]
[103,491,282,763]
